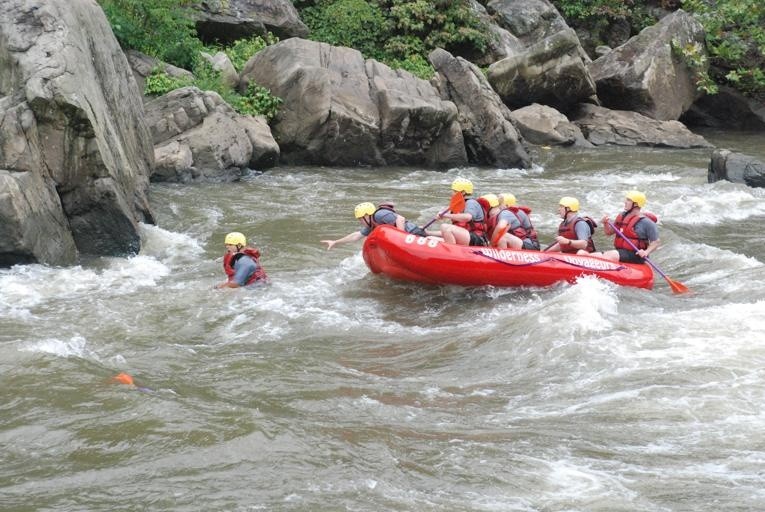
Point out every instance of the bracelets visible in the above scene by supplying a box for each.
[568,239,572,246]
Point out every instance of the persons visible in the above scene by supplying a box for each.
[589,190,660,263]
[319,202,428,252]
[419,178,541,251]
[545,197,595,255]
[213,231,270,288]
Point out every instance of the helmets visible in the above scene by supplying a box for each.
[354,202,376,219]
[559,196,580,212]
[224,231,247,248]
[451,178,473,197]
[498,193,517,207]
[625,191,647,208]
[483,193,499,208]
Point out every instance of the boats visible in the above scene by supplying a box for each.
[360,224,656,289]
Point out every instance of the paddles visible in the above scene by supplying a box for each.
[606,220,690,295]
[492,223,512,247]
[423,192,465,229]
